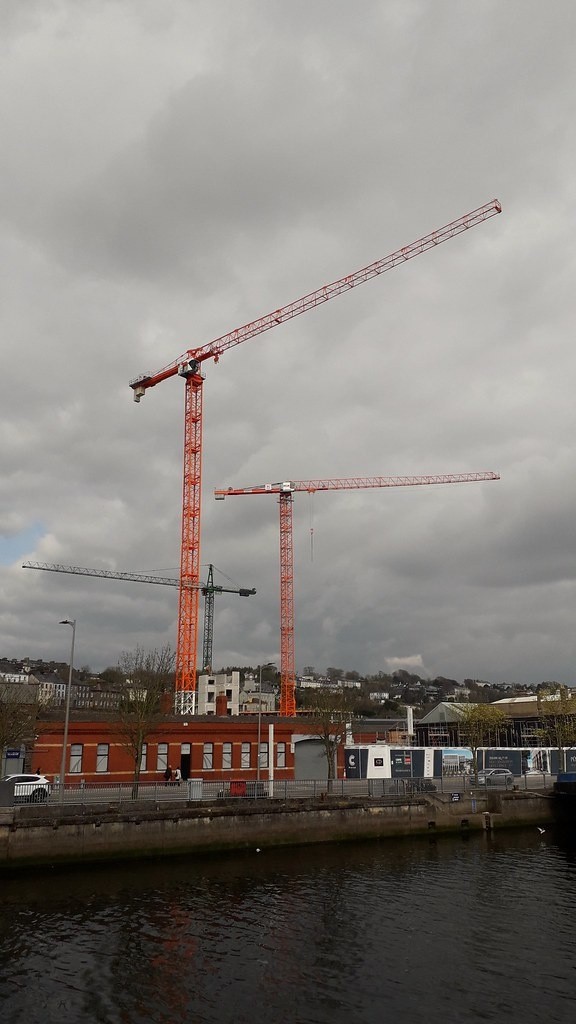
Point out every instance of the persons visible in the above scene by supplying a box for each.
[173,767,181,786]
[165,765,173,786]
[36,768,42,775]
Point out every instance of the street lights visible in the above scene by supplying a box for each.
[57,619,77,806]
[257,662,276,781]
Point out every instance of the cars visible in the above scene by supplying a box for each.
[1,774,53,803]
[469,769,514,787]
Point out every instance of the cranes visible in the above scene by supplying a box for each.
[213,472,500,716]
[129,199,503,716]
[22,561,257,676]
